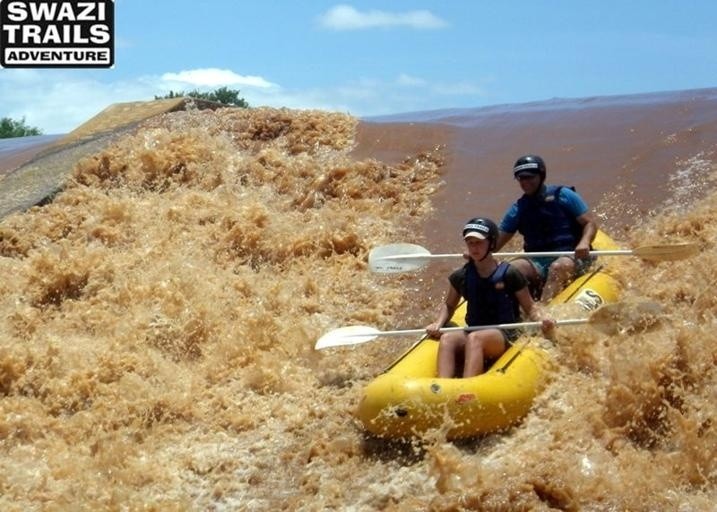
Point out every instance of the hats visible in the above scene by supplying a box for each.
[518,171,534,177]
[465,229,487,240]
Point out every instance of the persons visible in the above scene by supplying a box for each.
[463,156,596,305]
[426,216,553,379]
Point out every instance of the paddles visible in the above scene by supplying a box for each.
[314,301,663,351]
[368,242,699,274]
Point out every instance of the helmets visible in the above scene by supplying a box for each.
[462,217,499,243]
[514,156,546,178]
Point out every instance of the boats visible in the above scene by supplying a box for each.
[353,228,629,441]
